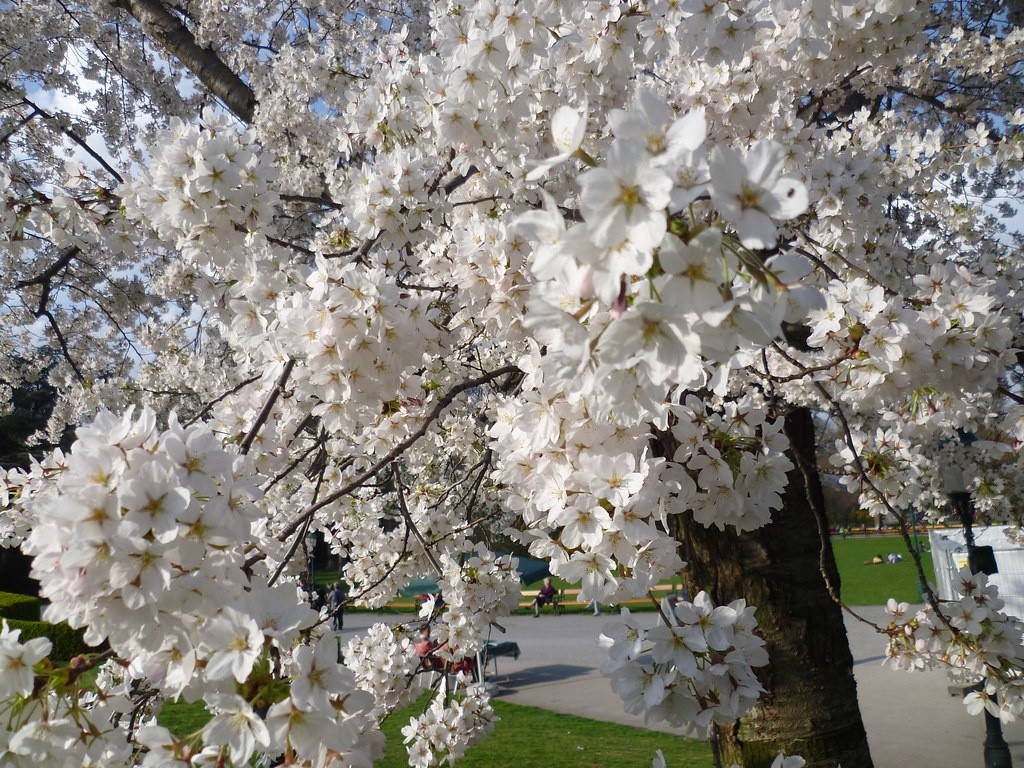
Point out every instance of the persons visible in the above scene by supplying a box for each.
[835,522,928,567]
[585,598,601,616]
[416,624,470,675]
[524,576,557,619]
[327,581,344,632]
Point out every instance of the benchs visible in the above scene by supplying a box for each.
[557,589,592,613]
[675,584,684,602]
[519,590,562,615]
[614,584,674,614]
[380,594,418,615]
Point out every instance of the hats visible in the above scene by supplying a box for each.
[313,584,321,591]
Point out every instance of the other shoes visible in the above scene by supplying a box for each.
[525,607,530,610]
[533,615,539,618]
[332,623,343,631]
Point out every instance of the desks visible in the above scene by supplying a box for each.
[472,640,521,683]
